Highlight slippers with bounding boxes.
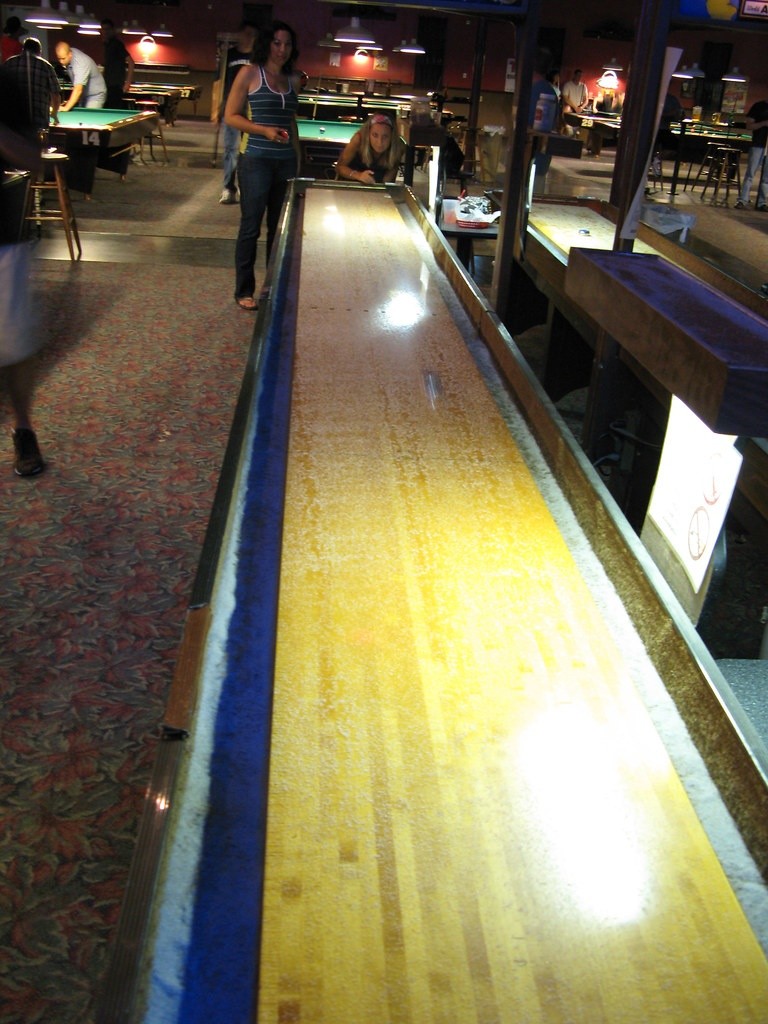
[235,293,257,310]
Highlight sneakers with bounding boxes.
[11,427,43,477]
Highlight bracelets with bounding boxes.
[350,171,356,180]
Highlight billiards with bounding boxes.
[691,129,694,132]
[320,127,325,133]
[704,131,707,134]
[617,116,620,119]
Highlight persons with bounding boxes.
[592,89,619,158]
[528,70,589,126]
[0,69,48,477]
[225,22,302,311]
[216,21,258,203]
[100,19,133,108]
[335,112,406,185]
[652,94,679,163]
[4,36,60,202]
[0,16,28,62]
[56,42,107,113]
[736,100,768,211]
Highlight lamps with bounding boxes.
[317,33,342,48]
[602,56,624,71]
[672,65,693,79]
[392,40,407,53]
[687,63,705,78]
[116,20,147,35]
[355,25,383,51]
[721,67,746,82]
[24,0,101,35]
[334,17,375,44]
[400,39,425,54]
[151,24,174,38]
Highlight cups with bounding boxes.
[342,84,349,93]
[336,83,342,92]
[712,113,722,123]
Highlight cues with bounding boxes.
[312,63,324,120]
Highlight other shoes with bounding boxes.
[218,188,235,205]
[651,153,662,178]
[733,199,751,209]
[754,205,768,212]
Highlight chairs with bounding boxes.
[433,137,481,196]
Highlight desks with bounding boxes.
[435,196,498,269]
[48,107,160,201]
[295,93,453,183]
[395,116,445,188]
[56,78,203,126]
[565,112,755,164]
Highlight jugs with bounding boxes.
[693,106,702,122]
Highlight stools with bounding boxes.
[121,98,170,166]
[25,153,82,264]
[683,142,741,206]
